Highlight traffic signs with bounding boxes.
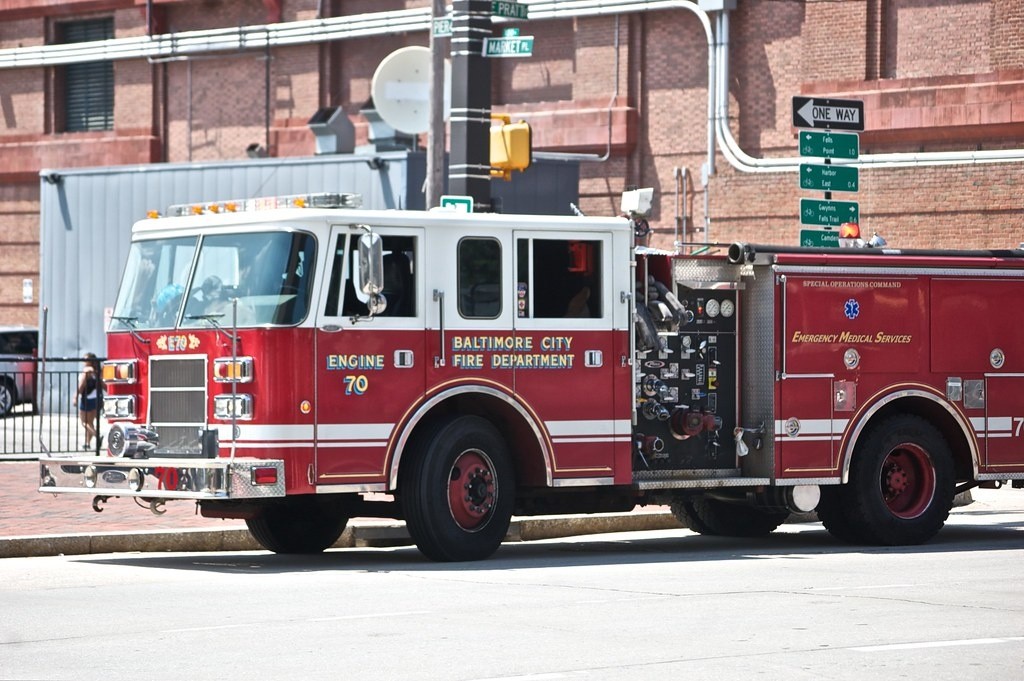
[793,97,865,248]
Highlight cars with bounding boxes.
[0,327,38,416]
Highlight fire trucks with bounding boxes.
[36,193,1024,566]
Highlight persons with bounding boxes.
[167,275,228,321]
[71,353,107,450]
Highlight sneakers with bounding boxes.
[99,435,104,447]
[83,444,90,451]
